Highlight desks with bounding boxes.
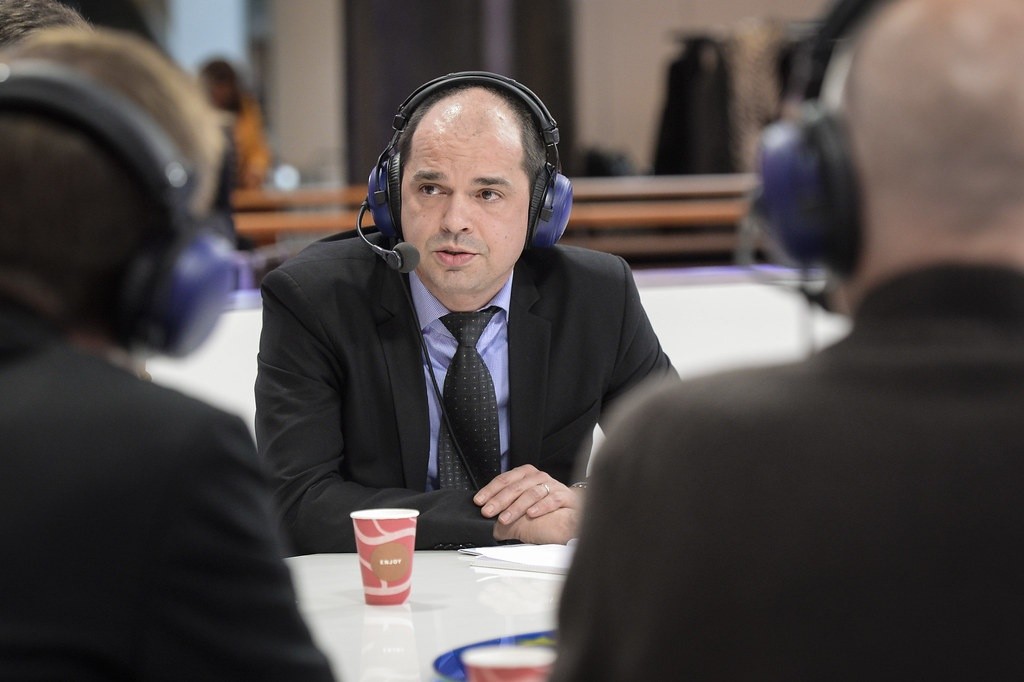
[284,551,566,682]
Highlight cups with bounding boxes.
[349,508,420,606]
[461,643,557,682]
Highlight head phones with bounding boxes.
[367,71,573,250]
[0,55,255,359]
[747,0,875,281]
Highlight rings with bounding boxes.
[537,483,549,493]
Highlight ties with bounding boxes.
[436,306,501,491]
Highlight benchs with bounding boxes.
[148,265,848,471]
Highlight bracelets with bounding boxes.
[579,485,587,488]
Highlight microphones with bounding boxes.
[737,226,829,313]
[357,196,420,273]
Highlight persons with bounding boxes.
[202,62,269,189]
[255,70,682,551]
[0,28,337,681]
[659,39,734,175]
[556,0,1024,682]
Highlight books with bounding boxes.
[469,538,578,576]
